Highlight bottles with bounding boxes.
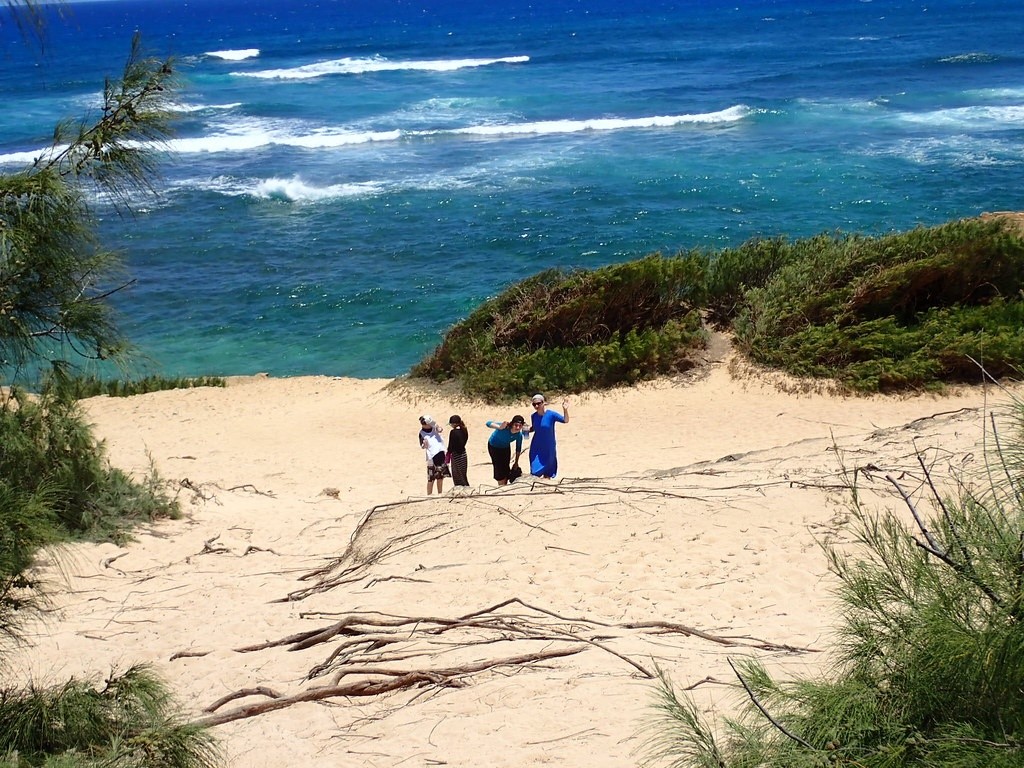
[445,454,450,463]
[523,428,529,439]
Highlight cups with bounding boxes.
[423,440,428,448]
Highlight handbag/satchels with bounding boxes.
[510,466,522,483]
[433,451,445,466]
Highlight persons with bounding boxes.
[525,394,570,478]
[418,415,452,495]
[444,414,469,486]
[485,415,525,486]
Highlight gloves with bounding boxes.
[446,452,451,463]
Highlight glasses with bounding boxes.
[532,401,542,406]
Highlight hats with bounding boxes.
[512,415,524,424]
[419,415,436,427]
